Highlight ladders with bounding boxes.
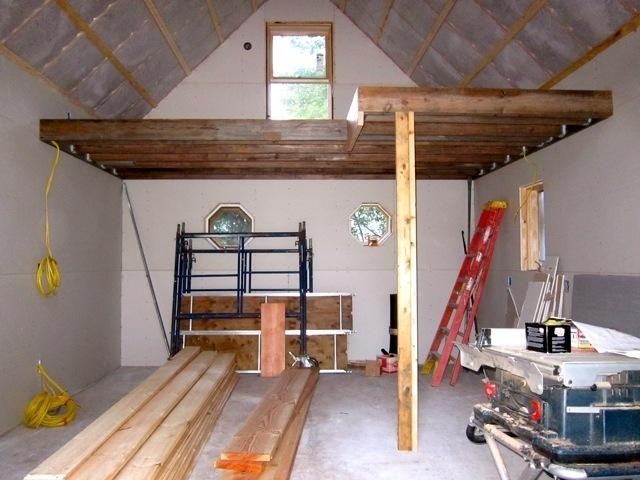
[419,201,508,388]
